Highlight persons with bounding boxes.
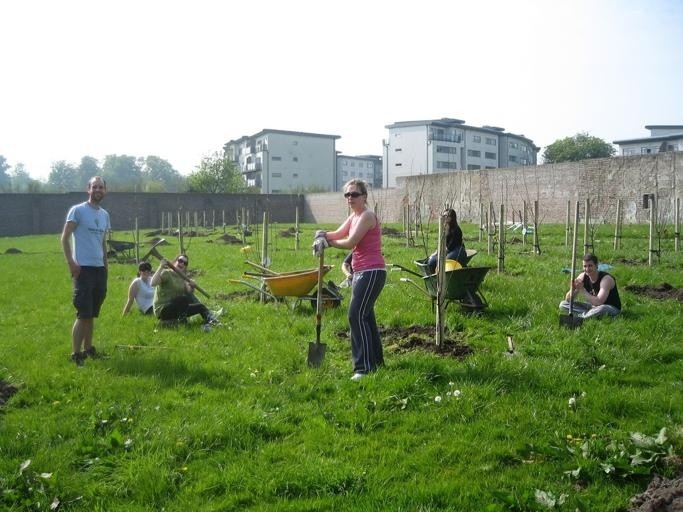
[61,176,111,364]
[340,251,354,288]
[149,255,223,325]
[122,261,156,316]
[431,208,467,268]
[312,178,386,380]
[559,252,621,320]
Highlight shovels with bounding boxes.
[307,233,327,369]
[558,200,584,330]
[501,333,520,361]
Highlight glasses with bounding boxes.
[178,258,188,266]
[345,192,363,198]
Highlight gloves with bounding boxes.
[313,231,329,257]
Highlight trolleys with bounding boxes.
[389,263,492,314]
[106,239,150,260]
[226,258,342,312]
